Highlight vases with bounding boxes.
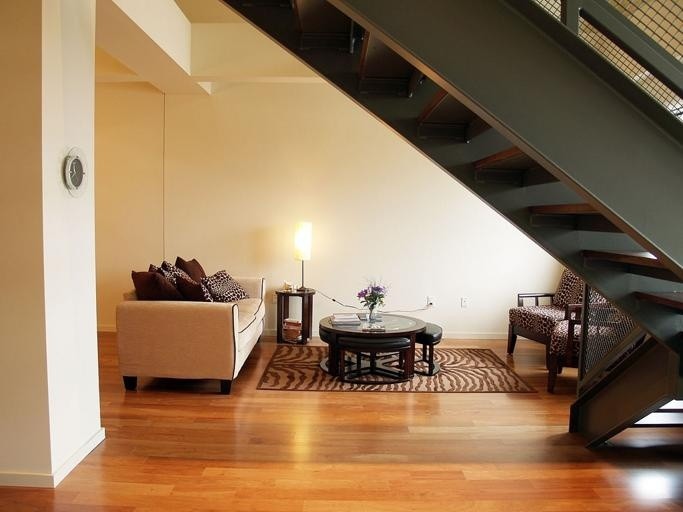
[366,309,376,323]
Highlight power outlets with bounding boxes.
[427,296,439,310]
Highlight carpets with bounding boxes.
[256,345,539,392]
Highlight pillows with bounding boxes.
[132,256,249,303]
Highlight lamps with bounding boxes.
[294,222,311,291]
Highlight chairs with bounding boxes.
[547,306,638,393]
[507,267,591,374]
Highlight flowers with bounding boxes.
[356,283,387,319]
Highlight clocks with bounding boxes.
[64,146,89,198]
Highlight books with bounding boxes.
[328,312,362,327]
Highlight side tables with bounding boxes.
[275,288,315,345]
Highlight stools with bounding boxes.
[339,336,411,384]
[396,323,442,376]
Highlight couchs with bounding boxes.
[116,278,265,395]
